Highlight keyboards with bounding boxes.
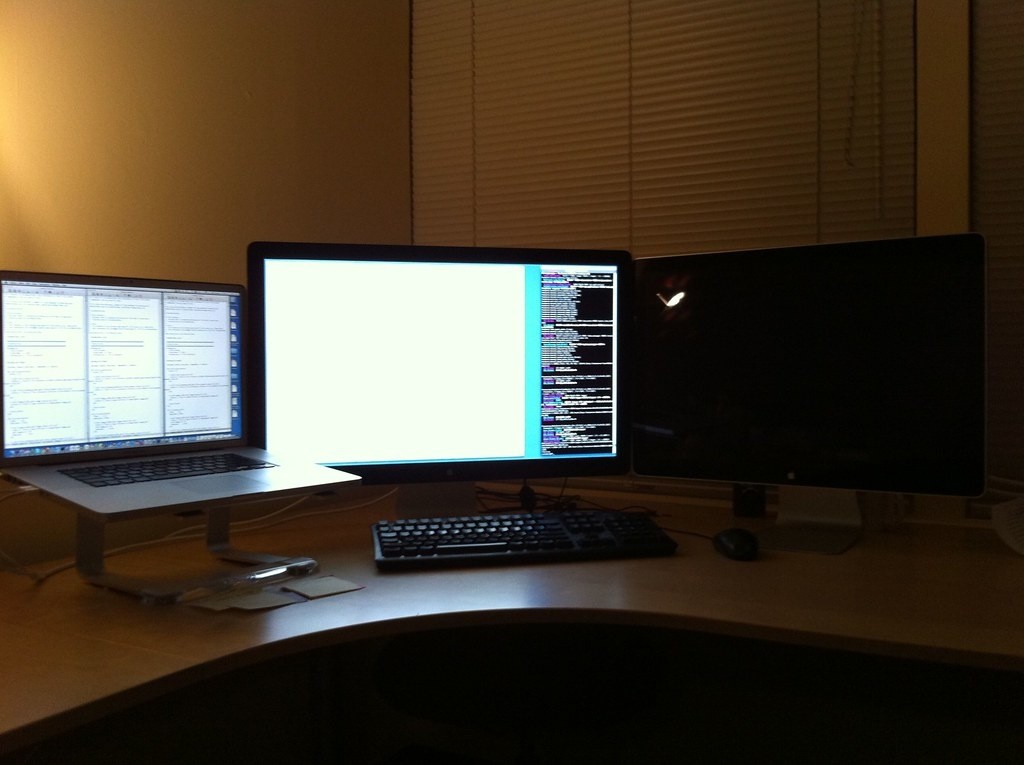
[371,513,675,568]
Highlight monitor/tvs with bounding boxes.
[245,240,634,520]
[627,233,988,557]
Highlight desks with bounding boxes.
[0,482,1023,754]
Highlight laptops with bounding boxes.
[0,270,360,524]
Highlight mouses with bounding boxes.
[713,528,760,560]
